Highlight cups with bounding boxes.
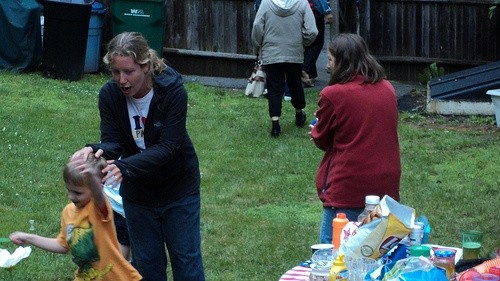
[311,249,376,281]
[462,232,483,260]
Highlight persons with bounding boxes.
[72,32,204,281]
[251,0,318,136]
[301,0,333,87]
[309,34,401,243]
[9,153,143,281]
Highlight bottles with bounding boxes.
[329,254,349,281]
[358,195,380,224]
[332,213,349,251]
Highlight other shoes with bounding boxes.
[271,119,280,137]
[300,71,316,88]
[295,109,307,127]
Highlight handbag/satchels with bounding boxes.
[245,67,265,99]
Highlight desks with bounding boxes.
[279,245,464,281]
